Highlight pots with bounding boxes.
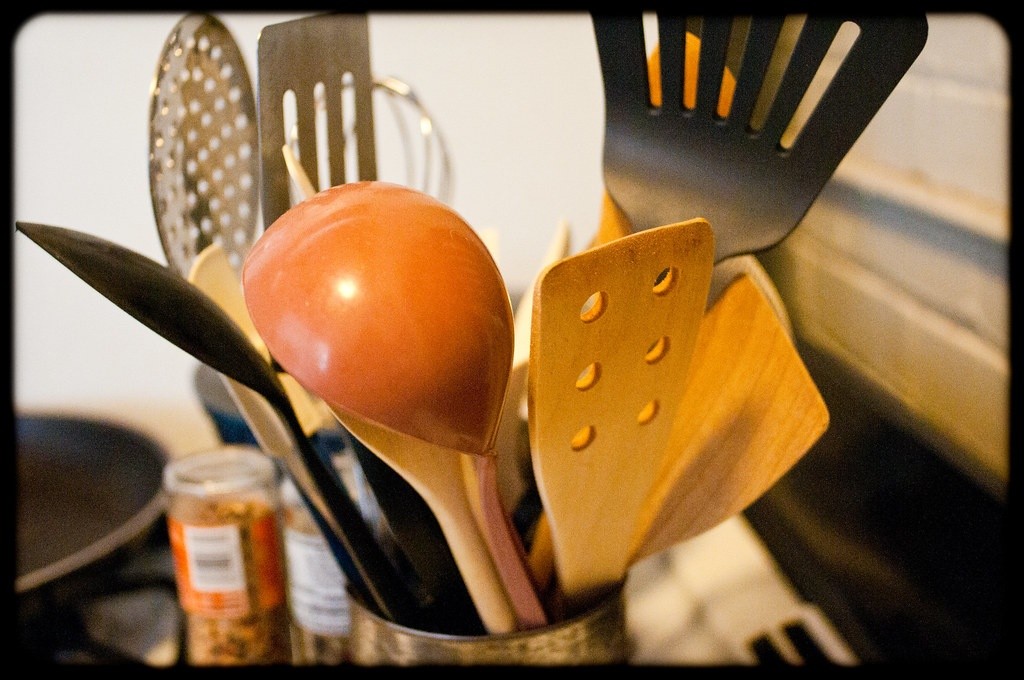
[17,415,169,666]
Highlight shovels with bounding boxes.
[12,9,930,637]
